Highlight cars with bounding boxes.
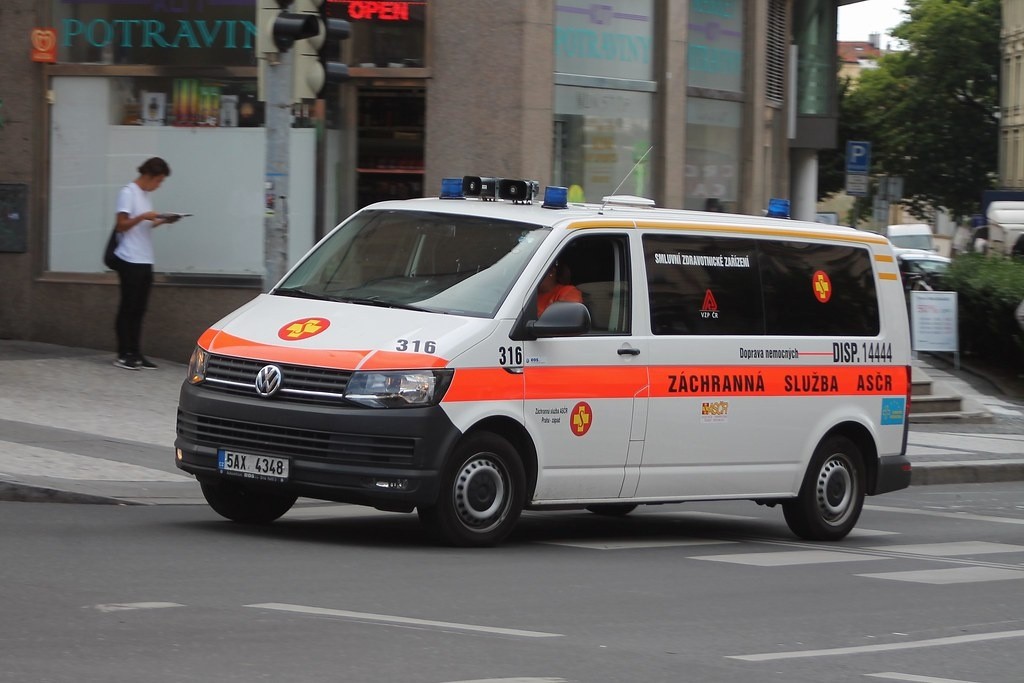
[896,253,951,301]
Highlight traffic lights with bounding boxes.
[297,14,351,131]
[255,0,319,63]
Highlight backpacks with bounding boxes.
[103,229,123,271]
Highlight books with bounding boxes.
[156,213,194,218]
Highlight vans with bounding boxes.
[174,177,913,540]
[887,224,938,254]
[986,201,1024,260]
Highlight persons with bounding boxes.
[114,157,181,370]
[537,260,583,317]
[702,197,722,213]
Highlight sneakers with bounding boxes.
[139,359,159,370]
[112,358,141,370]
